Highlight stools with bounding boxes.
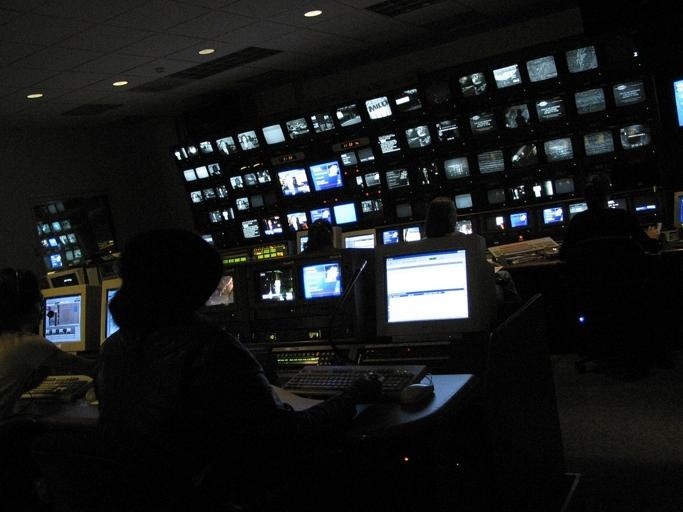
[1,412,50,512]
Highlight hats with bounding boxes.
[108,224,224,334]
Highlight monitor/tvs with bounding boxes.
[46,266,86,289]
[525,52,560,86]
[98,238,125,262]
[490,62,524,94]
[620,123,654,151]
[32,197,92,273]
[99,278,121,349]
[672,192,683,229]
[573,87,607,115]
[611,78,647,108]
[582,128,615,156]
[372,234,504,345]
[172,81,590,253]
[194,249,372,338]
[456,71,490,100]
[667,77,683,128]
[564,44,599,77]
[602,189,665,227]
[38,285,102,355]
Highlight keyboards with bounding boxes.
[20,374,94,402]
[486,237,561,260]
[282,364,428,402]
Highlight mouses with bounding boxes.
[400,383,434,409]
[85,387,96,401]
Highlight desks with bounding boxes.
[502,258,565,267]
[660,248,683,254]
[0,374,477,426]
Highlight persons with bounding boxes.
[0,267,98,512]
[559,175,660,261]
[298,219,349,258]
[417,196,513,306]
[95,225,381,512]
